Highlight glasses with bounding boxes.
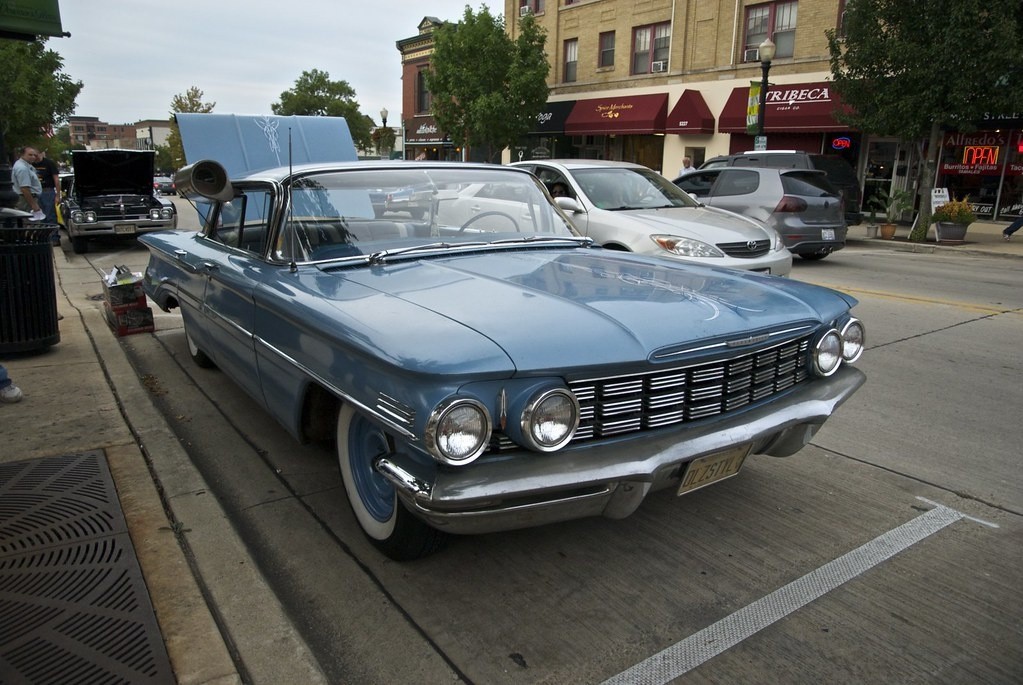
[551,190,566,196]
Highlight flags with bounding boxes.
[40,123,56,139]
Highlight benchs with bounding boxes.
[244,218,413,257]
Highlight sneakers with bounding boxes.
[0,384,24,404]
[1003,230,1010,241]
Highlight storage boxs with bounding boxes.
[102,301,154,337]
[102,272,146,310]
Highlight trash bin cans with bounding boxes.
[0,222,62,359]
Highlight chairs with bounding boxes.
[285,222,353,261]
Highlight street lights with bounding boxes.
[156,151,160,168]
[380,107,388,128]
[758,38,776,135]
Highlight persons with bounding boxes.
[11,146,42,223]
[32,146,61,247]
[0,364,22,403]
[552,181,569,199]
[596,177,628,208]
[1002,215,1023,241]
[678,157,696,178]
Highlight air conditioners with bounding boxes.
[744,48,761,63]
[651,60,668,72]
[520,6,531,16]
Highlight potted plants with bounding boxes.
[870,188,919,239]
[866,204,880,238]
[930,192,980,239]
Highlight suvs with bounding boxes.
[697,149,864,225]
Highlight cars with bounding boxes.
[135,111,867,562]
[645,167,847,261]
[154,176,177,196]
[436,158,792,278]
[55,173,74,228]
[61,149,178,255]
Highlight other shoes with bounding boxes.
[52,239,61,246]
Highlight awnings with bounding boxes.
[665,89,715,136]
[406,115,453,144]
[519,92,669,134]
[718,65,1023,137]
